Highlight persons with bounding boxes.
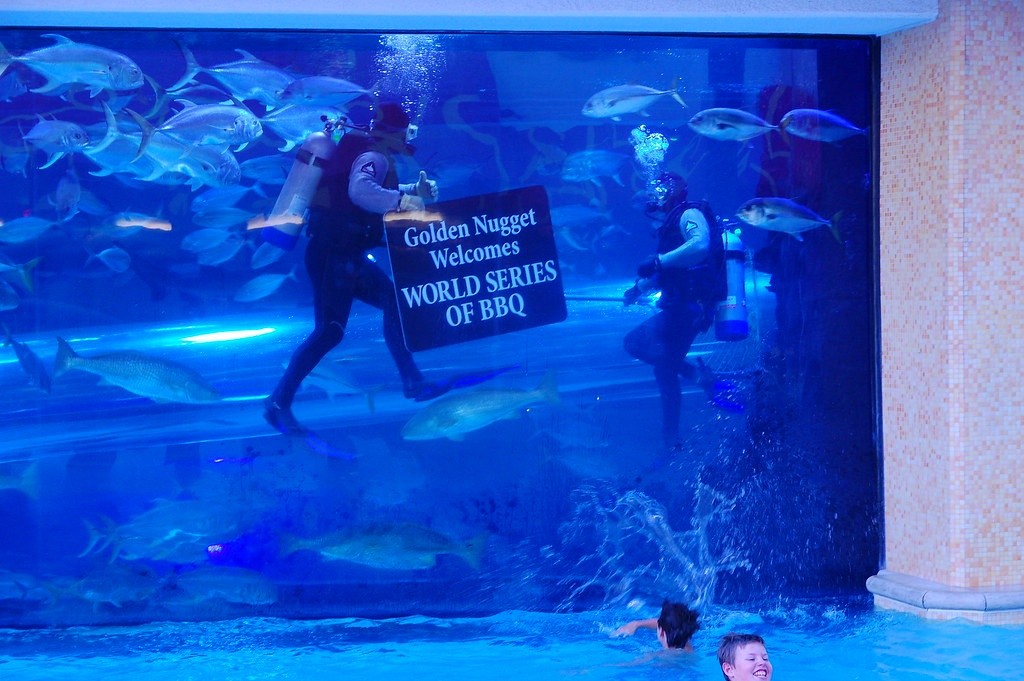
[623,172,729,453]
[263,103,447,433]
[717,633,772,681]
[615,601,700,652]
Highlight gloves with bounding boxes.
[624,285,642,307]
[417,171,439,203]
[399,193,425,216]
[637,255,662,281]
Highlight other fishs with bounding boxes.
[1,500,285,614]
[400,367,563,445]
[0,32,379,305]
[1,321,226,405]
[277,515,487,573]
[550,70,872,252]
